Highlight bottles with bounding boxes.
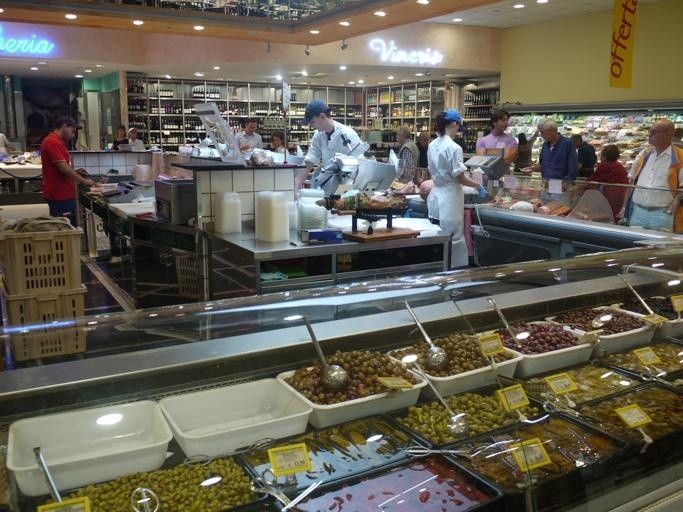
[128,100,147,111]
[149,133,196,143]
[134,80,144,93]
[151,102,195,114]
[157,86,173,98]
[219,104,279,115]
[230,120,242,130]
[464,93,490,105]
[129,118,204,131]
[464,107,492,118]
[228,88,238,99]
[193,88,220,99]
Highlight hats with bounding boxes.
[445,109,464,133]
[54,115,83,131]
[301,99,330,125]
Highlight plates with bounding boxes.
[41,454,268,512]
[600,336,683,380]
[672,335,681,343]
[236,413,432,494]
[559,379,683,458]
[657,372,682,392]
[516,359,653,413]
[440,412,631,508]
[236,495,287,511]
[278,448,503,511]
[384,383,553,450]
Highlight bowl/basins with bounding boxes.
[159,378,314,464]
[613,296,683,337]
[545,305,662,356]
[276,356,429,428]
[8,398,173,497]
[386,335,523,400]
[473,320,600,379]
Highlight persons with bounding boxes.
[536,119,577,180]
[517,126,538,167]
[235,117,262,151]
[393,127,420,183]
[294,99,360,199]
[577,144,628,219]
[416,131,430,169]
[272,132,290,155]
[570,134,597,177]
[128,128,145,151]
[40,116,95,228]
[476,110,518,166]
[616,119,683,231]
[113,125,128,150]
[427,109,480,270]
[0,132,9,154]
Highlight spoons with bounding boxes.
[592,311,613,329]
[303,315,347,392]
[414,362,470,437]
[404,300,448,371]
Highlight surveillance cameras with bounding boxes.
[341,43,348,50]
[304,49,311,56]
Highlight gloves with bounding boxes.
[478,185,488,199]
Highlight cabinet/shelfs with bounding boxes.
[462,83,499,120]
[460,121,491,152]
[399,172,683,262]
[498,102,683,178]
[2,242,683,511]
[366,82,444,157]
[127,78,363,152]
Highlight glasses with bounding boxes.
[647,129,657,136]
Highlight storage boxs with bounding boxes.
[153,180,194,224]
[0,284,87,362]
[171,247,212,300]
[1,219,83,293]
